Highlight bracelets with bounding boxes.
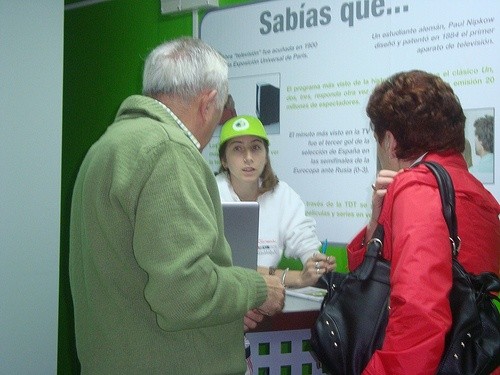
[361,238,366,247]
[282,268,289,284]
[269,266,275,275]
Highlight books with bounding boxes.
[286,285,327,302]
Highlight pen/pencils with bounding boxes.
[320,238,331,256]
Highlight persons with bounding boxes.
[467,115,494,184]
[346,69,500,375]
[214,115,337,289]
[69,36,287,375]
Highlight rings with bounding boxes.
[313,253,318,259]
[315,262,320,268]
[371,184,377,190]
[316,269,320,274]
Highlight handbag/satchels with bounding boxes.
[313,161,499,375]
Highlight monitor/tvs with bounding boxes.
[221,202,261,272]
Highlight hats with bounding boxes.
[217,115,269,151]
